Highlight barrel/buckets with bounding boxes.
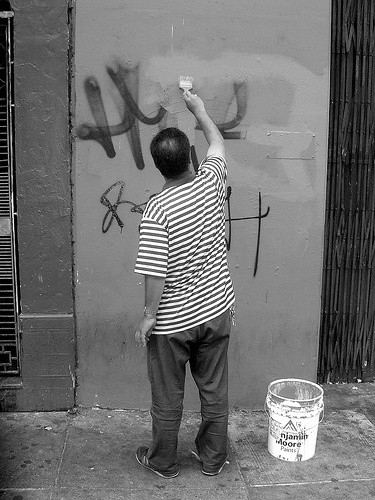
[264,378,325,463]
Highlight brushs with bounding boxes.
[178,75,195,96]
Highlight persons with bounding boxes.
[134,92,235,479]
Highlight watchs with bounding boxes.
[144,306,158,314]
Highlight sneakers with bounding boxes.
[202,457,229,475]
[135,445,179,478]
